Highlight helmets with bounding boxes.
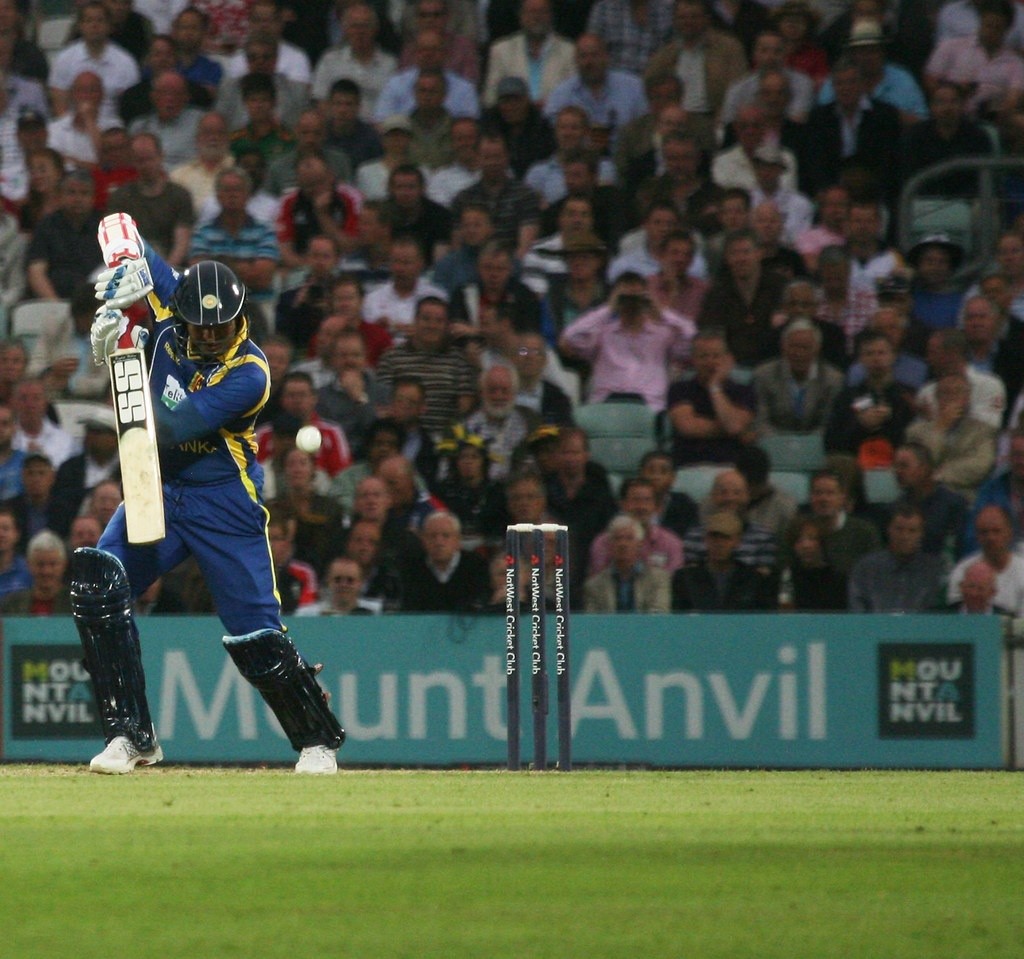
[172,261,246,327]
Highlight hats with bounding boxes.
[838,16,893,48]
[376,112,412,136]
[78,406,118,431]
[705,513,744,538]
[901,226,968,267]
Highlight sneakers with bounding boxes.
[293,743,340,776]
[88,723,165,773]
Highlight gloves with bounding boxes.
[89,301,151,370]
[96,257,154,310]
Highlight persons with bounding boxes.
[0,0,1024,618]
[68,213,346,775]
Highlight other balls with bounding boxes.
[296,426,322,453]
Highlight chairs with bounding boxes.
[571,403,829,516]
[909,196,976,262]
